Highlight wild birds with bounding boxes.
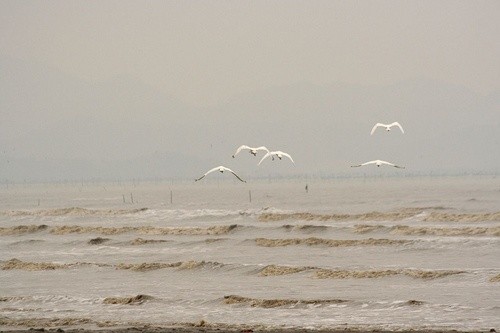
[370,121,405,134]
[194,166,247,184]
[232,144,296,167]
[350,159,406,170]
